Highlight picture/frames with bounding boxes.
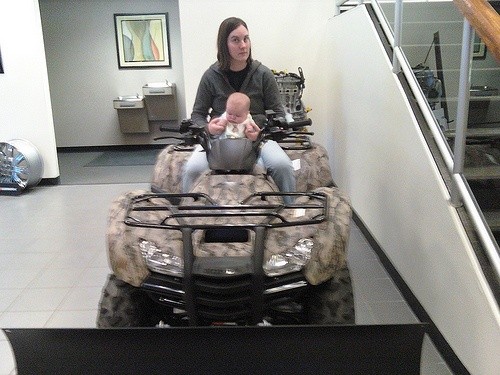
[114,12,172,69]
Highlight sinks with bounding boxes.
[142,83,175,95]
[113,96,144,109]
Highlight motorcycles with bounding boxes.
[95,68,357,328]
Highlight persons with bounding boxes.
[213,92,258,140]
[182,17,296,205]
